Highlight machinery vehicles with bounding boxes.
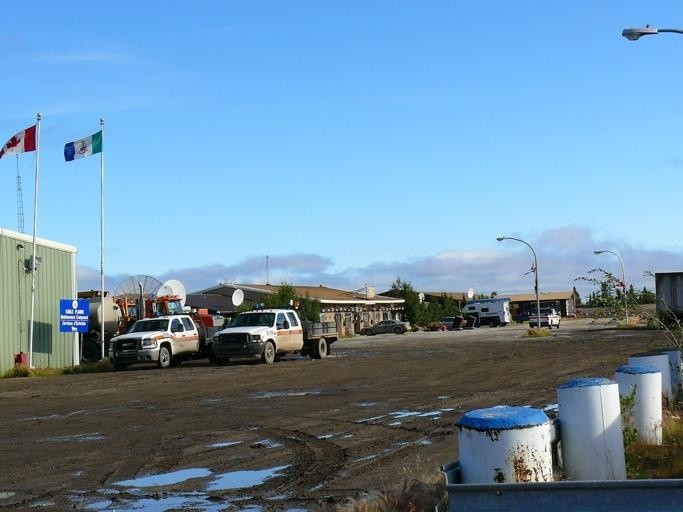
[77,291,225,368]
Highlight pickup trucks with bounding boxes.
[209,308,338,364]
[528,308,561,329]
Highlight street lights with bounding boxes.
[594,250,629,323]
[621,24,683,41]
[497,237,541,329]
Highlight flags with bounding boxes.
[0,125,37,158]
[64,130,102,161]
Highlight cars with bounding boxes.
[431,317,466,327]
[362,319,411,335]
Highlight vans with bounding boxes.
[461,298,511,328]
